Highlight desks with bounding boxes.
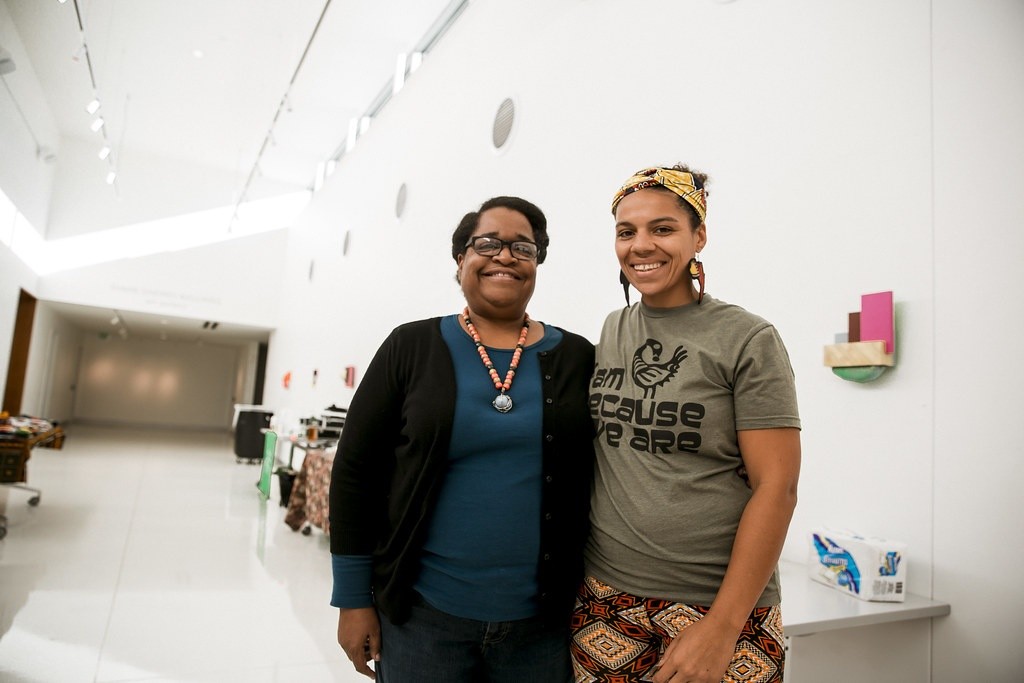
[779,560,951,683]
[0,412,67,538]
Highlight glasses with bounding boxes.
[465,235,541,261]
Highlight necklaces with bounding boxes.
[463,305,530,414]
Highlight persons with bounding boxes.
[568,165,802,682]
[331,196,594,683]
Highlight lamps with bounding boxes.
[90,117,104,132]
[72,44,88,61]
[106,172,116,184]
[85,99,101,115]
[99,145,111,160]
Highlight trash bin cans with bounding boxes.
[278,467,298,507]
